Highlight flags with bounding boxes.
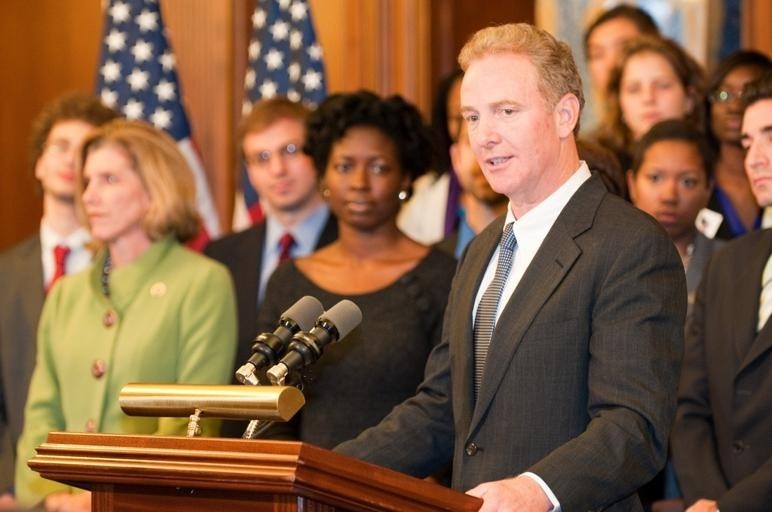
[93,0,220,254]
[232,1,328,239]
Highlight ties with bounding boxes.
[473,222,518,404]
[278,234,296,265]
[46,245,70,294]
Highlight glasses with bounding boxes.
[707,87,772,102]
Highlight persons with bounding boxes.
[330,22,688,512]
[699,51,768,239]
[14,118,238,512]
[600,37,707,148]
[264,91,458,489]
[394,65,464,222]
[0,95,126,512]
[673,77,766,512]
[584,5,663,101]
[400,109,511,257]
[201,98,338,438]
[623,119,727,312]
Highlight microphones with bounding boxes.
[266,300,362,384]
[235,296,325,383]
